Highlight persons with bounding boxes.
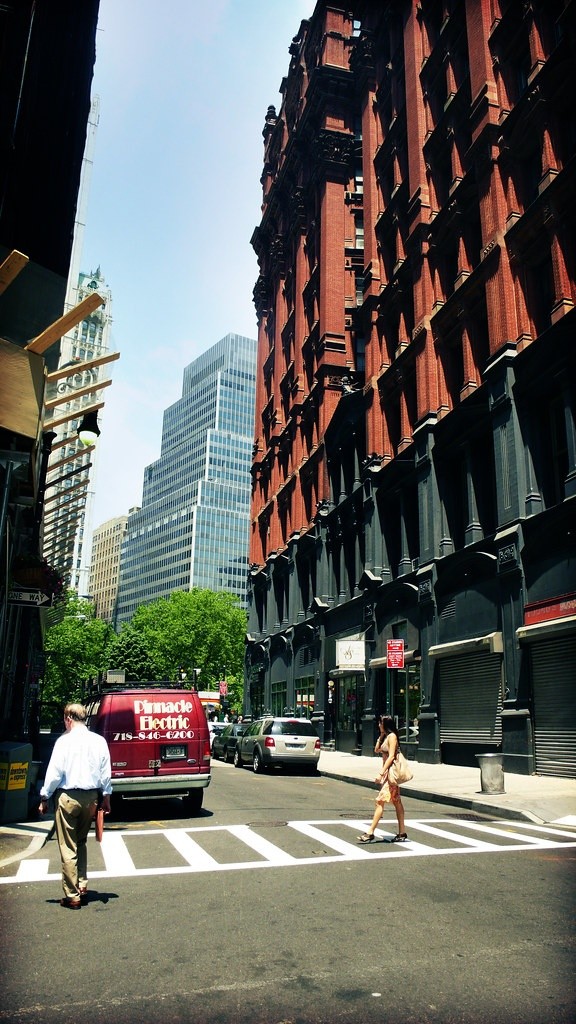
[237,716,243,724]
[38,702,113,909]
[223,714,229,723]
[356,715,408,842]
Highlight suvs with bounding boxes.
[81,669,211,819]
[234,718,321,774]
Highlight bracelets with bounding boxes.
[380,773,384,776]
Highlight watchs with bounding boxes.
[42,799,48,803]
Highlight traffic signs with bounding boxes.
[5,587,54,608]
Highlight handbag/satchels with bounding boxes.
[388,751,413,786]
[96,808,104,842]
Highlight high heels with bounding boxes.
[391,833,407,842]
[356,833,374,843]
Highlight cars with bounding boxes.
[208,722,251,763]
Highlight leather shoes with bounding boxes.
[79,887,87,895]
[60,898,81,909]
